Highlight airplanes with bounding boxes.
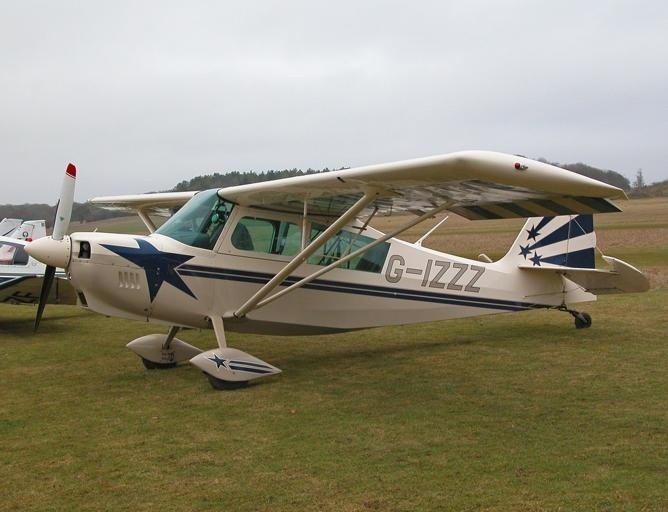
[1,216,76,307]
[1,148,654,390]
